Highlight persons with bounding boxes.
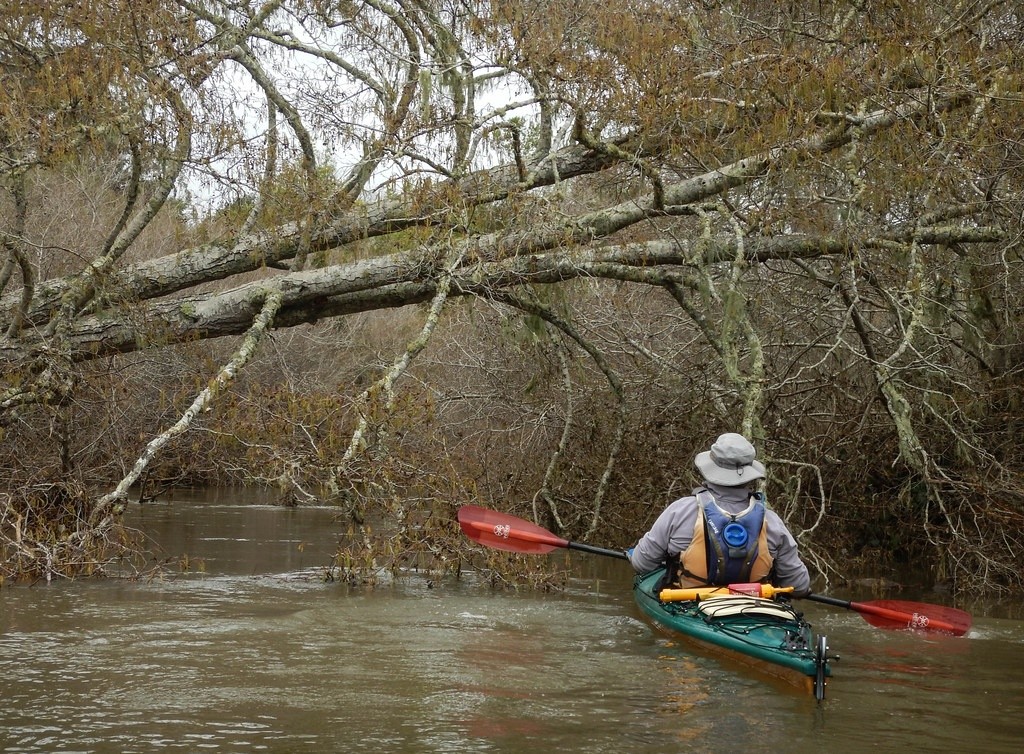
[626,431,811,607]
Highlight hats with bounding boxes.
[695,433,766,486]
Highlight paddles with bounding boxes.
[454,503,973,640]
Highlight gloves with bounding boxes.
[624,549,634,563]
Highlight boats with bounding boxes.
[632,566,844,702]
